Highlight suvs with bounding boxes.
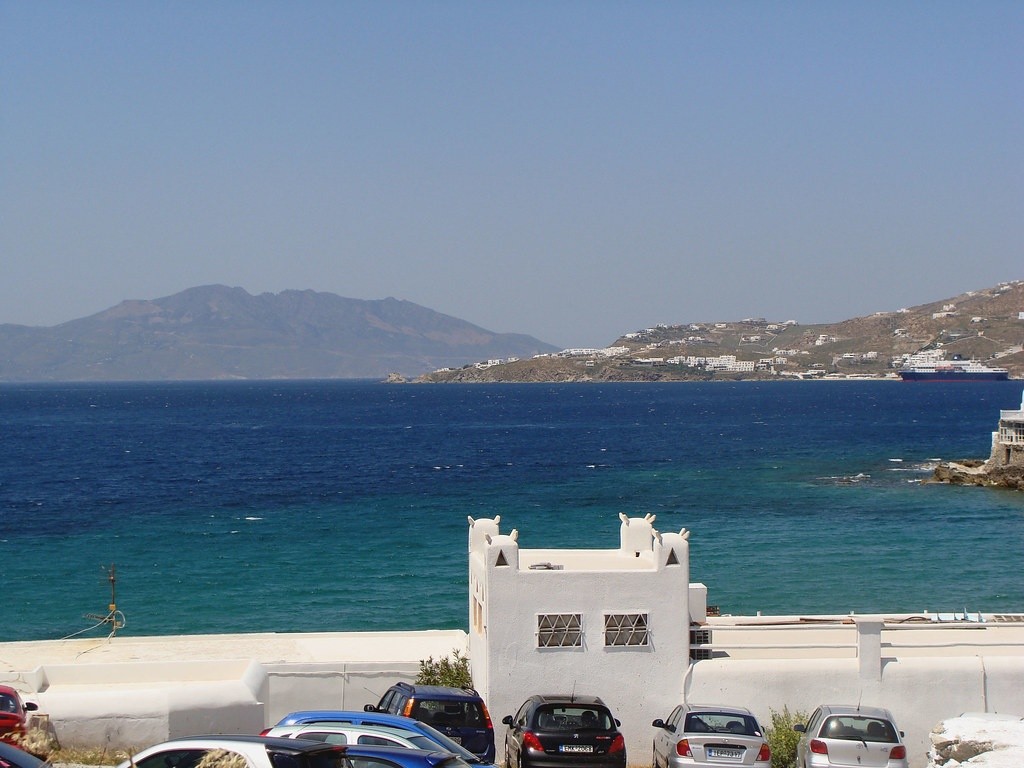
[363,681,496,764]
[502,693,627,768]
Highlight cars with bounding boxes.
[258,723,453,754]
[337,742,473,768]
[0,684,55,768]
[113,732,355,768]
[793,703,908,768]
[652,702,773,768]
[260,709,504,768]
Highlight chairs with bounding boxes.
[688,718,707,730]
[538,711,555,729]
[829,720,843,737]
[868,722,882,737]
[726,720,742,728]
[418,707,432,718]
[580,710,598,726]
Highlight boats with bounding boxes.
[897,354,1009,382]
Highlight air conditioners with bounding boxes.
[691,629,712,660]
